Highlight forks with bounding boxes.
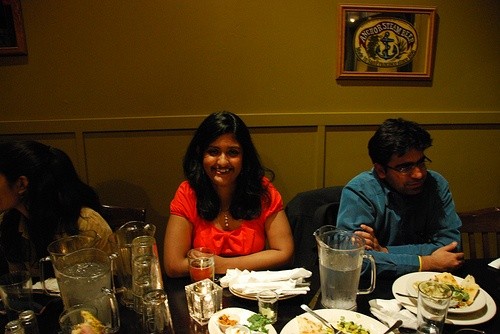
[300,304,347,334]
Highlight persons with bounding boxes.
[0,139,125,276]
[165,110,293,278]
[333,118,464,283]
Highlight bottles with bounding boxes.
[18,309,40,334]
[4,319,26,334]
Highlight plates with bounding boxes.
[422,288,486,314]
[208,307,277,334]
[228,281,307,300]
[391,272,497,325]
[279,308,395,334]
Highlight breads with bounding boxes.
[298,316,325,334]
[435,273,458,288]
[461,275,479,306]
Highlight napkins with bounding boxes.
[368,299,427,329]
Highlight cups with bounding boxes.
[58,304,98,334]
[416,280,454,334]
[0,270,33,314]
[140,289,175,334]
[187,247,216,284]
[131,235,164,315]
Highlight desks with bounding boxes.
[0,259,500,334]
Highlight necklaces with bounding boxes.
[220,210,230,227]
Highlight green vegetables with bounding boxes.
[243,314,271,334]
[337,321,371,334]
[439,284,469,300]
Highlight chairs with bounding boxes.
[286,186,343,268]
[101,205,146,233]
[457,208,500,258]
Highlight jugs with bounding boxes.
[313,225,377,311]
[38,229,102,297]
[108,221,157,309]
[52,248,121,334]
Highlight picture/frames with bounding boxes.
[335,3,436,81]
[0,0,28,56]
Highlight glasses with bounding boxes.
[386,155,433,174]
